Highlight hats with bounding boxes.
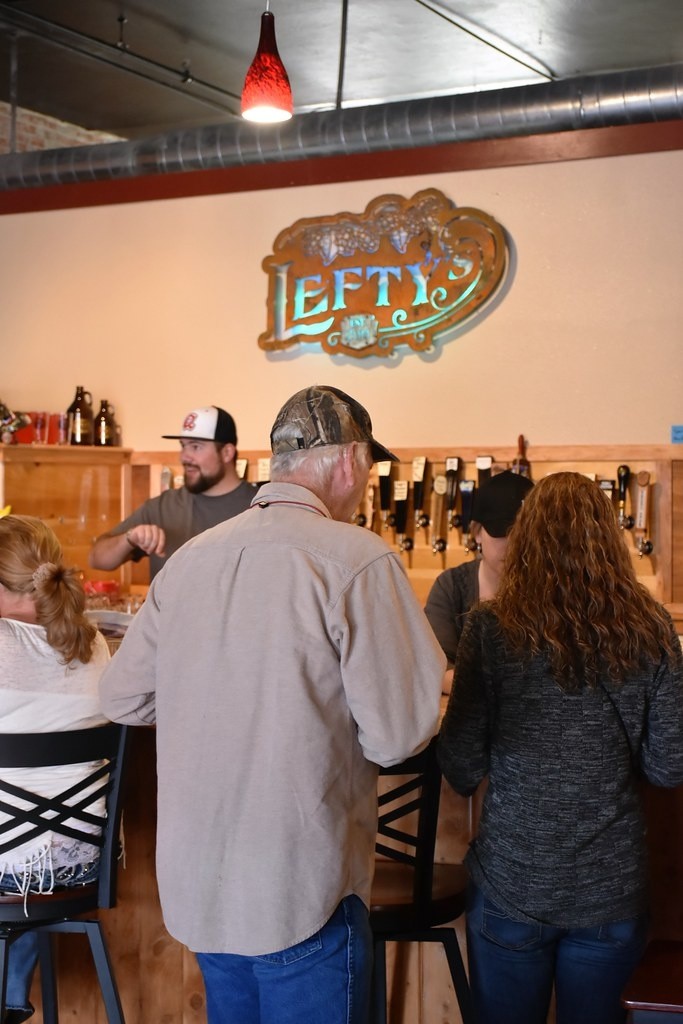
[160,405,238,447]
[269,385,401,465]
[470,470,537,538]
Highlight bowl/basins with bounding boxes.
[82,608,135,638]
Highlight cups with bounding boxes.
[14,409,61,445]
[81,589,144,616]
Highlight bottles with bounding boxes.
[65,385,94,446]
[94,398,115,448]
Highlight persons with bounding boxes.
[99,386,448,1024]
[0,514,125,1024]
[89,405,259,587]
[423,471,536,695]
[439,472,683,1024]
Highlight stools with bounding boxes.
[0,713,127,1024]
[354,729,499,1024]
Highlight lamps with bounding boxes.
[239,0,292,122]
